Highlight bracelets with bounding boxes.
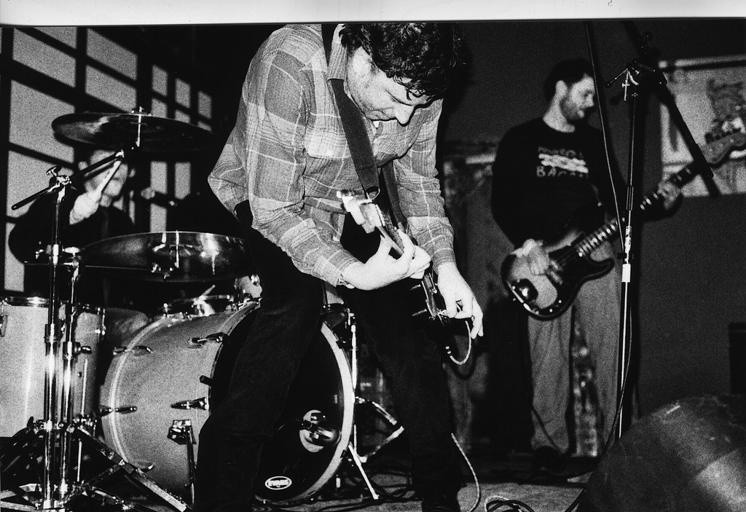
[522,241,538,257]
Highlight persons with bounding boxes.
[8,143,147,297]
[491,57,682,485]
[193,21,485,512]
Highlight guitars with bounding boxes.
[336,189,478,381]
[501,130,742,321]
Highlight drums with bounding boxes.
[97,304,356,511]
[0,289,105,437]
[578,394,742,512]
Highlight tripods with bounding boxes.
[0,144,192,512]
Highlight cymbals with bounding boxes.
[76,232,269,282]
[52,113,222,161]
